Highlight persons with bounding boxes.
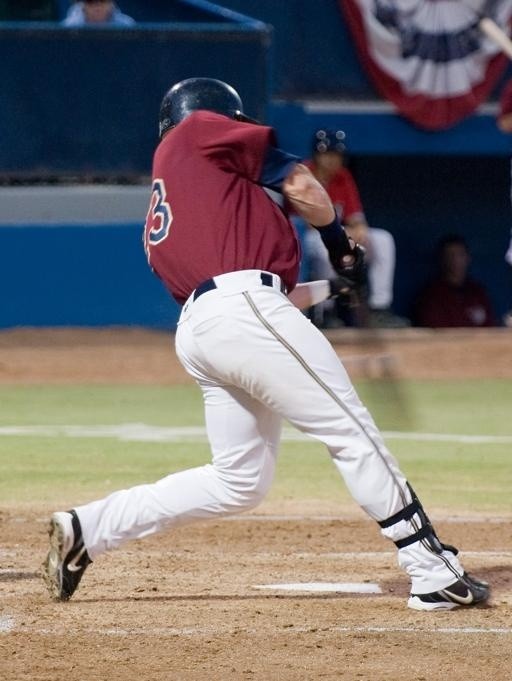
[282,128,411,329]
[413,233,493,327]
[59,2,156,184]
[496,75,512,133]
[46,76,494,611]
[500,242,512,327]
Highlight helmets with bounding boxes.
[157,76,261,141]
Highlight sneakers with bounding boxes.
[407,568,493,613]
[39,509,92,604]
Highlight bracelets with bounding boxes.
[311,207,341,241]
[309,279,331,305]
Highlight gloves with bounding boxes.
[327,276,372,306]
[324,226,366,276]
